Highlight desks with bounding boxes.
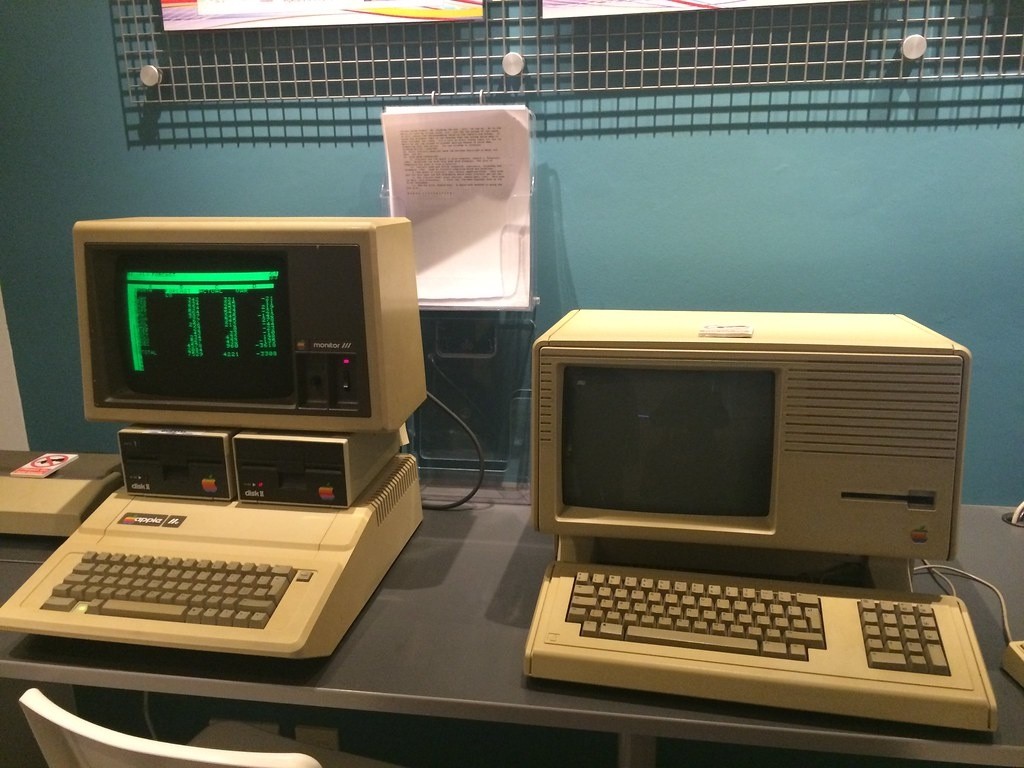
[0,485,1024,768]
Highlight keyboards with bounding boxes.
[37,549,314,631]
[566,573,951,677]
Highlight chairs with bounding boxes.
[18,687,323,768]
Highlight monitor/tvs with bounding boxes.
[558,365,776,518]
[108,249,292,403]
